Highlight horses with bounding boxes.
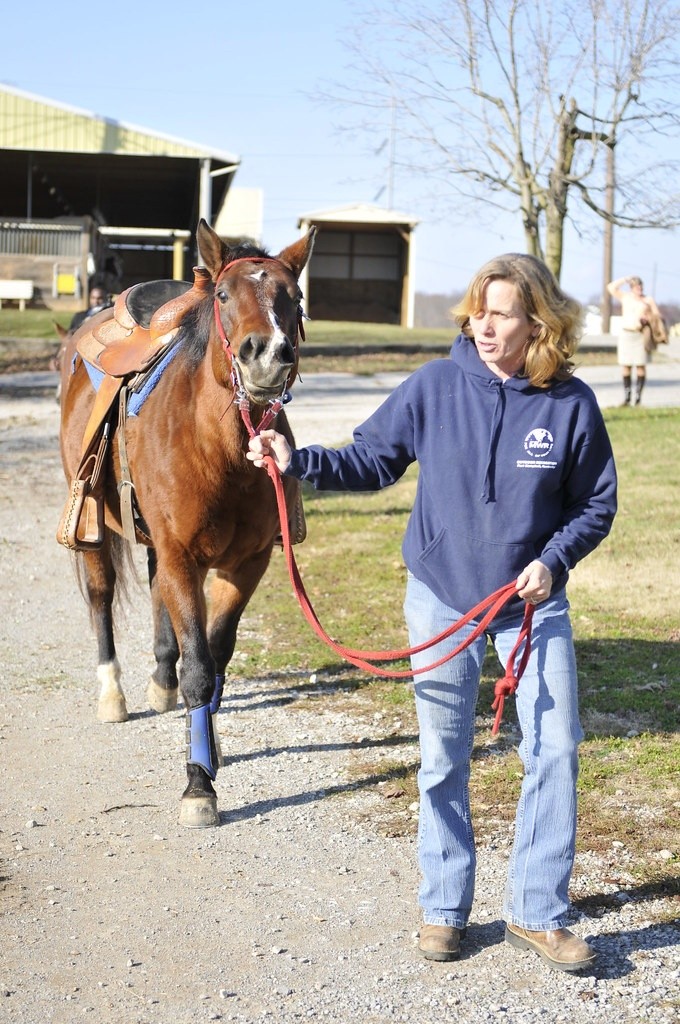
[58,222,322,826]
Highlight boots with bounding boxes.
[623,375,632,405]
[635,375,645,406]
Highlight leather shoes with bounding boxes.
[506,921,599,970]
[417,922,467,962]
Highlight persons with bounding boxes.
[244,252,618,975]
[70,271,120,334]
[606,274,668,405]
